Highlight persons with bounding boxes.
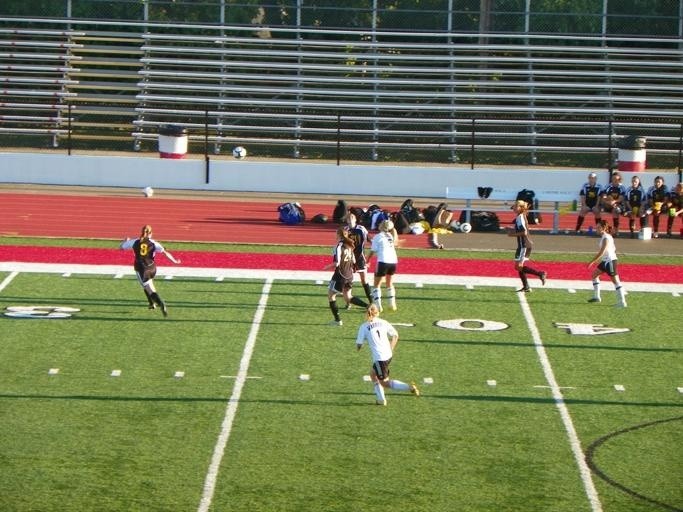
[575,173,683,238]
[506,200,545,293]
[355,303,420,407]
[586,220,627,309]
[120,225,180,317]
[326,213,398,326]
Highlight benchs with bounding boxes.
[445,186,611,234]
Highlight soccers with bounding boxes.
[450,220,460,231]
[233,146,247,160]
[460,222,472,233]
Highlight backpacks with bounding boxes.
[332,199,452,235]
[277,201,304,224]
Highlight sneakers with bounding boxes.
[538,270,546,285]
[408,381,419,396]
[613,302,626,307]
[375,400,386,405]
[665,234,670,238]
[587,298,601,303]
[652,231,658,238]
[615,233,624,237]
[328,319,342,326]
[517,287,532,293]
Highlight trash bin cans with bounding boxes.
[159,125,189,159]
[614,134,649,172]
